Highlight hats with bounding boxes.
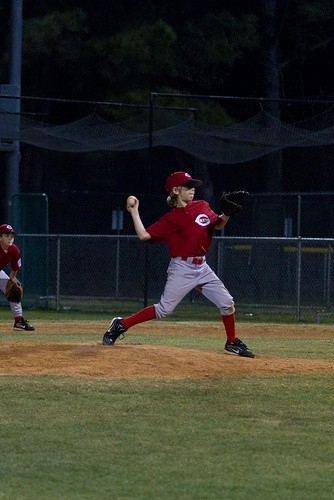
[0,224,16,235]
[165,171,203,192]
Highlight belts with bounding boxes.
[173,256,206,265]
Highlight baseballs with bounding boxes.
[128,196,137,206]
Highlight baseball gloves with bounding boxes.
[6,277,23,302]
[218,191,249,215]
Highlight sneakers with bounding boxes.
[103,317,127,345]
[13,317,35,332]
[224,337,255,358]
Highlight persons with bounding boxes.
[0,224,35,331]
[102,171,255,359]
